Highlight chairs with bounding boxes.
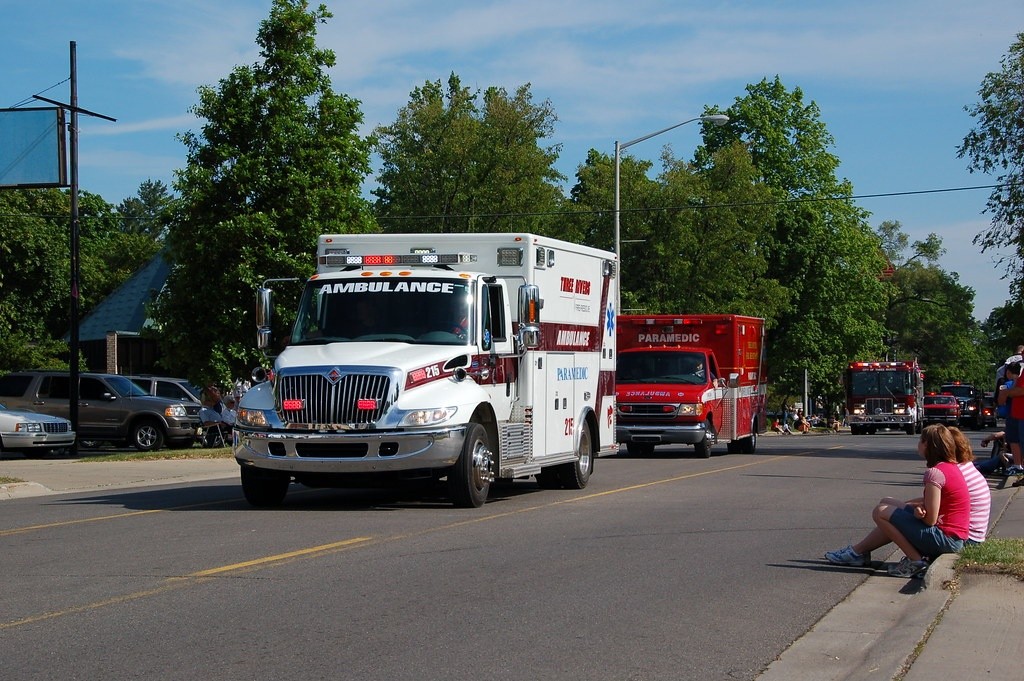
[201,422,227,448]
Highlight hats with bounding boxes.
[234,393,241,398]
[226,399,236,405]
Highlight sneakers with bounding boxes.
[824,544,871,566]
[888,557,930,578]
[1005,465,1024,476]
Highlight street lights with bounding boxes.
[615,112,729,313]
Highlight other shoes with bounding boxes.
[1012,478,1024,487]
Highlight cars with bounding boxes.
[982,394,999,427]
[0,403,77,460]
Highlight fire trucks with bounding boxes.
[939,381,984,430]
[843,356,925,435]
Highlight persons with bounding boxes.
[824,424,992,578]
[690,360,718,389]
[202,378,251,447]
[770,397,850,435]
[448,297,490,347]
[975,345,1024,487]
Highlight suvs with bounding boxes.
[922,394,964,430]
[1,371,204,452]
[115,374,206,408]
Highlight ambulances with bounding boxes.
[616,315,768,458]
[231,229,620,508]
